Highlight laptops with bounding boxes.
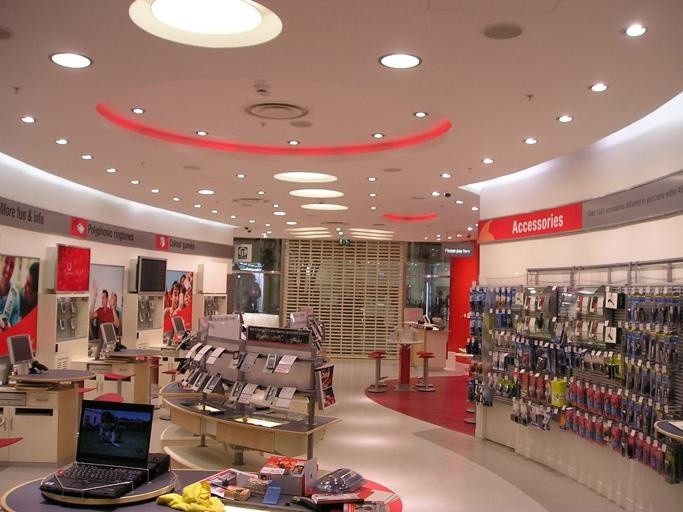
[41,400,154,498]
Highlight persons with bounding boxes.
[163,269,194,333]
[89,288,122,338]
[0,255,22,332]
[18,260,40,319]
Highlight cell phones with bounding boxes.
[292,465,304,474]
[262,487,280,505]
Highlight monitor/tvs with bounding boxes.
[7,334,34,364]
[171,316,186,333]
[100,322,118,344]
[135,256,167,295]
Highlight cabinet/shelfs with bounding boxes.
[158,334,343,466]
[463,278,682,485]
[0,344,196,467]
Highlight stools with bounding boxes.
[367,350,387,393]
[415,350,436,392]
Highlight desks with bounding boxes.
[0,467,258,512]
[386,339,423,392]
